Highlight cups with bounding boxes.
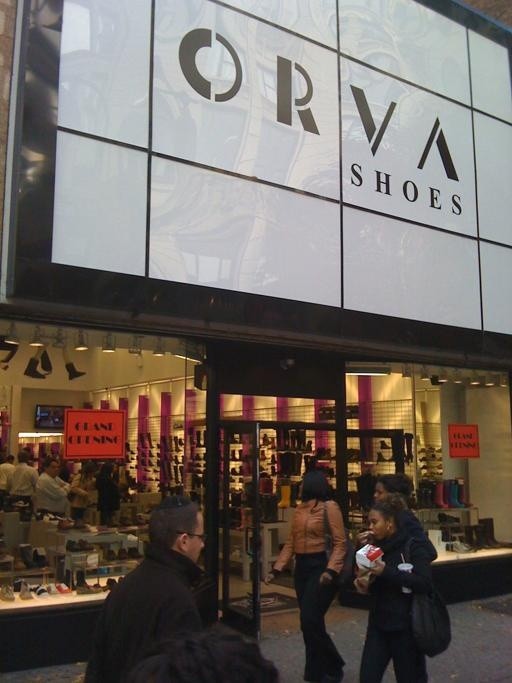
[397,562,416,595]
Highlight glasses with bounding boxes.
[175,531,207,541]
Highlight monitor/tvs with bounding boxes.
[35,404,73,429]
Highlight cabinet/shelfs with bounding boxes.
[0,574,132,673]
[338,507,512,610]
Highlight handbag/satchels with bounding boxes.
[322,507,354,584]
[410,587,452,661]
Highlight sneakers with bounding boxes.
[0,520,145,603]
[414,445,444,477]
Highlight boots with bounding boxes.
[24,351,52,380]
[124,427,414,509]
[66,362,86,381]
[436,513,504,552]
[414,478,471,510]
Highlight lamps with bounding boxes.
[402,366,507,387]
[5,321,200,363]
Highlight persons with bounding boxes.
[126,624,279,683]
[264,471,348,683]
[373,473,414,510]
[352,492,438,683]
[84,494,206,683]
[0,442,127,527]
